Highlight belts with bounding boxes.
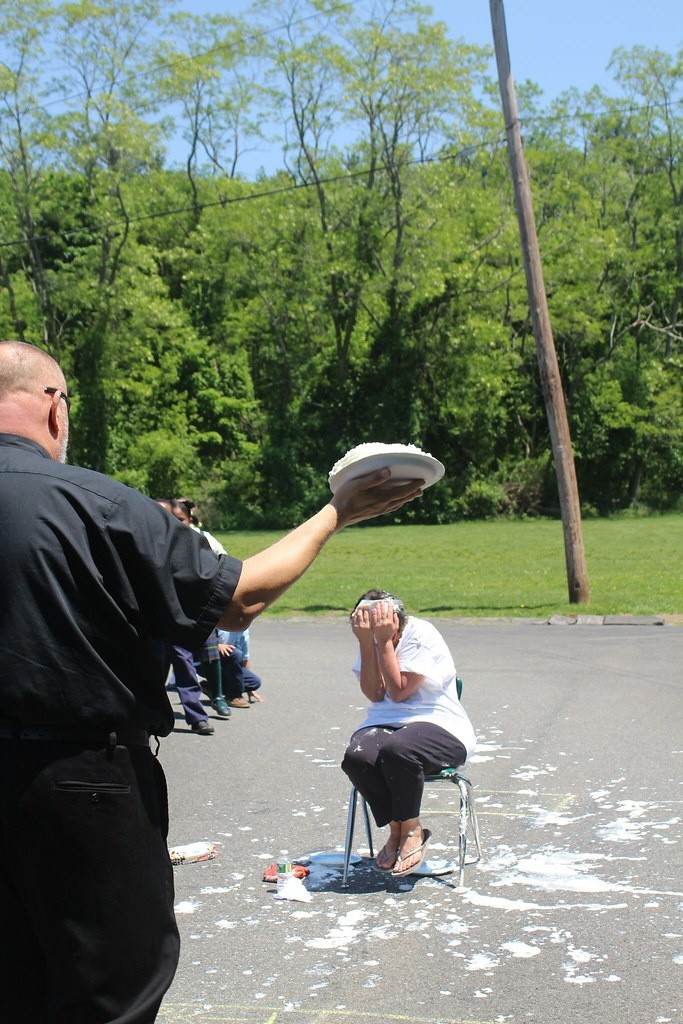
[0,720,149,746]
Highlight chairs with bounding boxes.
[340,677,481,889]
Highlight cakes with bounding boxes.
[328,442,435,483]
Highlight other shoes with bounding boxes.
[192,719,214,733]
[211,698,232,716]
[225,696,250,708]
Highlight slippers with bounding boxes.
[372,845,395,873]
[391,828,432,878]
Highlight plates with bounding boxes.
[411,860,455,875]
[309,853,362,865]
[330,452,445,495]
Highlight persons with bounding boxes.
[152,498,262,734]
[341,590,477,879]
[0,341,426,1024]
